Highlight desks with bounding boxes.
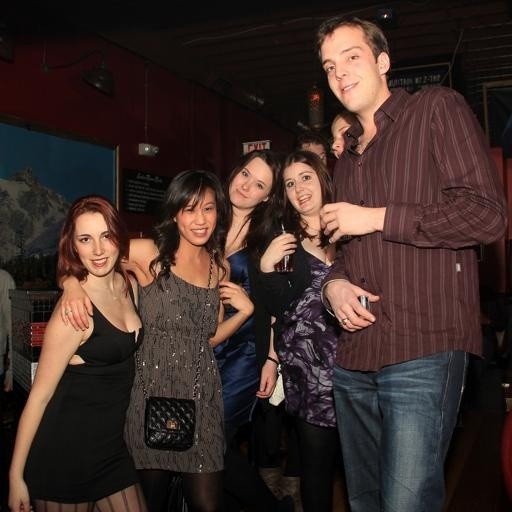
[9,288,62,393]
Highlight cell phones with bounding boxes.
[356,295,372,316]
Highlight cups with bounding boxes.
[273,230,296,273]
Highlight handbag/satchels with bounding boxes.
[142,394,197,453]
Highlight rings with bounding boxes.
[342,318,349,325]
[65,309,72,314]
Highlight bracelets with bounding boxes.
[267,356,278,364]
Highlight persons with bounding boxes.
[260,150,352,510]
[6,197,146,512]
[318,10,508,511]
[294,131,331,169]
[56,170,256,512]
[217,150,280,512]
[330,110,355,161]
